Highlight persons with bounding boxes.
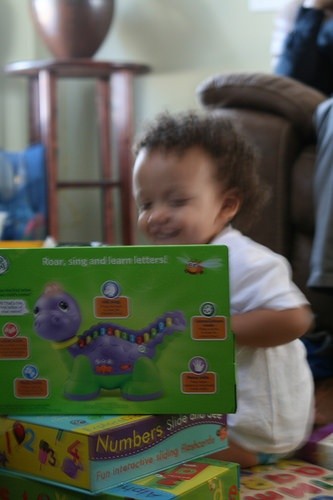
[129,107,318,467]
[278,1,333,290]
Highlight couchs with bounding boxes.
[201,72,333,329]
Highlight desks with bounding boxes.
[8,59,150,245]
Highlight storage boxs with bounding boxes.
[0,244,240,500]
